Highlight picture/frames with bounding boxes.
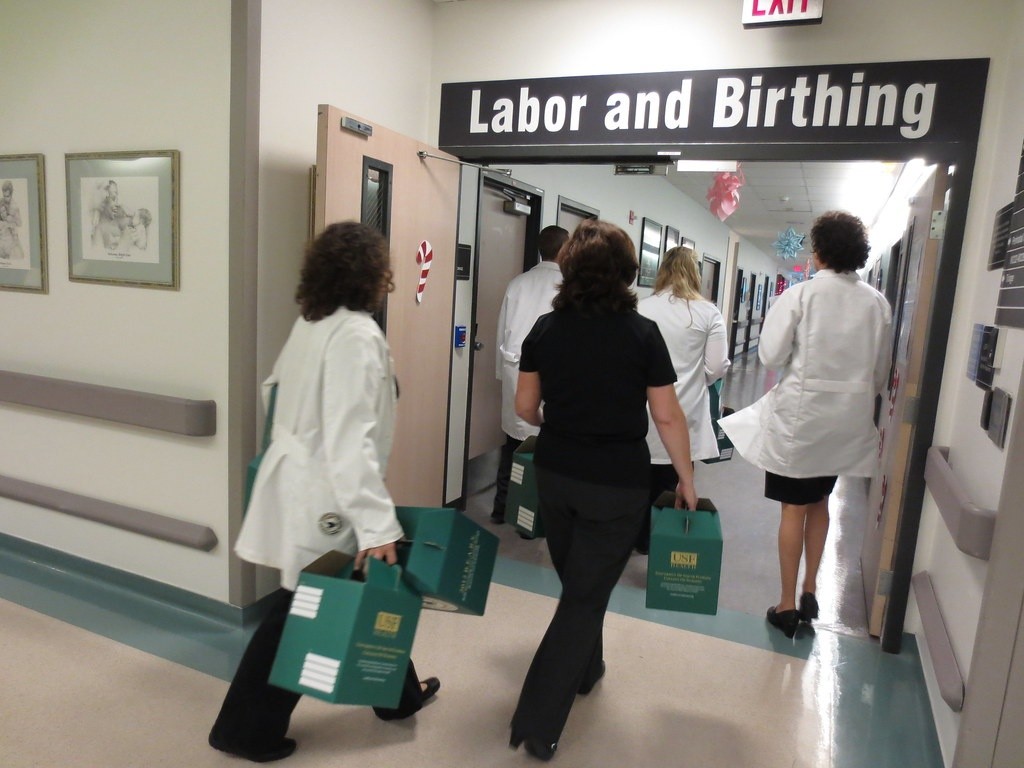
[637,216,696,288]
[64,148,182,293]
[0,152,50,296]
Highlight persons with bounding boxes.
[715,211,892,637]
[510,220,699,761]
[208,223,440,763]
[635,247,729,554]
[491,226,569,539]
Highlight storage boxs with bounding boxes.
[644,490,725,615]
[502,432,558,538]
[358,504,500,616]
[701,377,736,464]
[267,548,426,710]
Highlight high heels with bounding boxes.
[767,606,799,637]
[799,592,818,622]
[576,659,605,695]
[510,717,556,762]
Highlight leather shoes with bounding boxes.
[416,676,440,703]
[208,720,297,763]
